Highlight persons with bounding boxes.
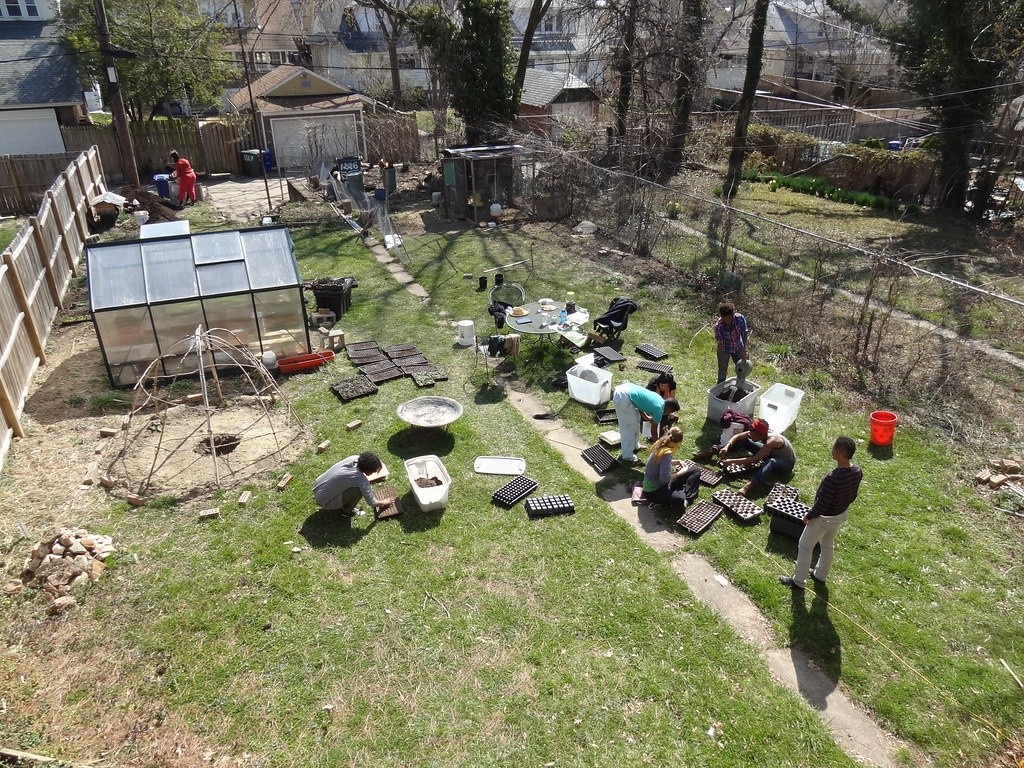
[613,373,680,466]
[778,436,864,589]
[716,418,797,495]
[313,452,395,517]
[641,426,701,508]
[714,303,751,384]
[170,149,198,207]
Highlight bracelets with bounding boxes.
[725,441,733,446]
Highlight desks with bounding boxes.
[506,302,588,354]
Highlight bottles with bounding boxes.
[561,309,567,323]
[566,292,576,313]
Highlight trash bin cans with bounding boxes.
[152,174,170,198]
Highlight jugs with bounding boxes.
[734,358,753,390]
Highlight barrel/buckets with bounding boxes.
[490,203,501,216]
[456,319,475,345]
[720,422,744,446]
[375,189,385,201]
[869,411,897,447]
[479,277,487,289]
[348,171,365,200]
[495,274,504,285]
[432,192,441,207]
[133,211,149,227]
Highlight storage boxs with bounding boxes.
[405,455,451,512]
[566,364,614,406]
[706,377,761,425]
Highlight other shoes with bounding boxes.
[640,445,647,450]
[778,574,804,592]
[622,455,638,462]
[340,507,365,518]
[809,568,825,585]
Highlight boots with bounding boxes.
[693,445,720,461]
[738,478,759,497]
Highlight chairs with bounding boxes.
[475,333,522,381]
[591,296,638,345]
[487,284,527,336]
